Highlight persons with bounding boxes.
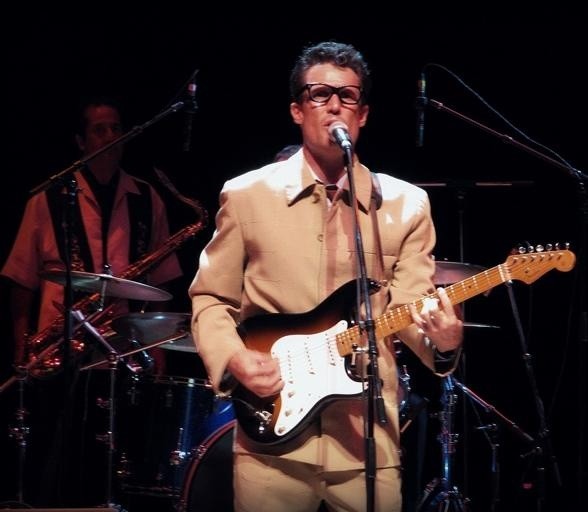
[187,40,464,512]
[0,95,184,503]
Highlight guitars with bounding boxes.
[232,242,577,444]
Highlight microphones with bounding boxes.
[327,121,352,150]
[417,71,426,148]
[183,79,196,151]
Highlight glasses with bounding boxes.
[294,83,363,105]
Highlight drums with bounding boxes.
[396,365,410,415]
[164,402,238,511]
[124,341,211,388]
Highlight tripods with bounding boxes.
[415,376,467,512]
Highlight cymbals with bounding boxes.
[112,310,198,353]
[430,261,488,286]
[36,269,173,302]
